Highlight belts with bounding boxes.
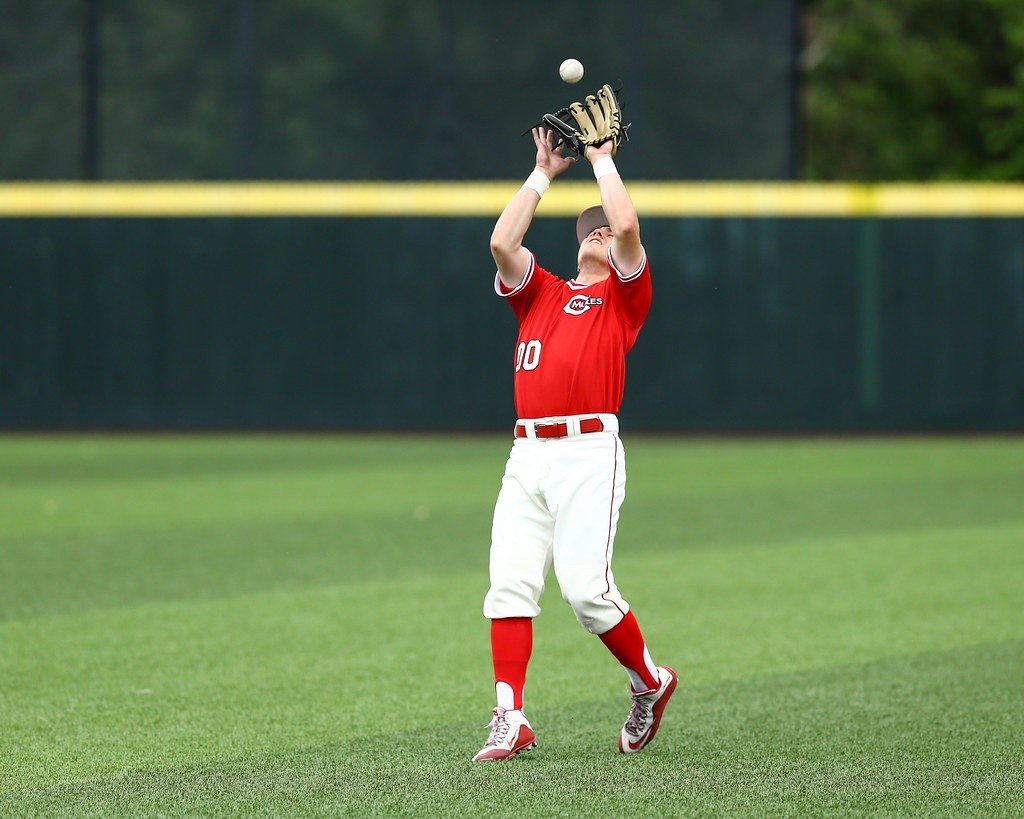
[515,418,603,438]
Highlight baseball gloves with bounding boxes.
[520,77,632,163]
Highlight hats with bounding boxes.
[576,205,609,245]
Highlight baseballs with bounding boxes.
[558,58,584,84]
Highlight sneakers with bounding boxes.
[472,708,536,761]
[618,665,679,754]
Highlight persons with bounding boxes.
[467,84,677,763]
